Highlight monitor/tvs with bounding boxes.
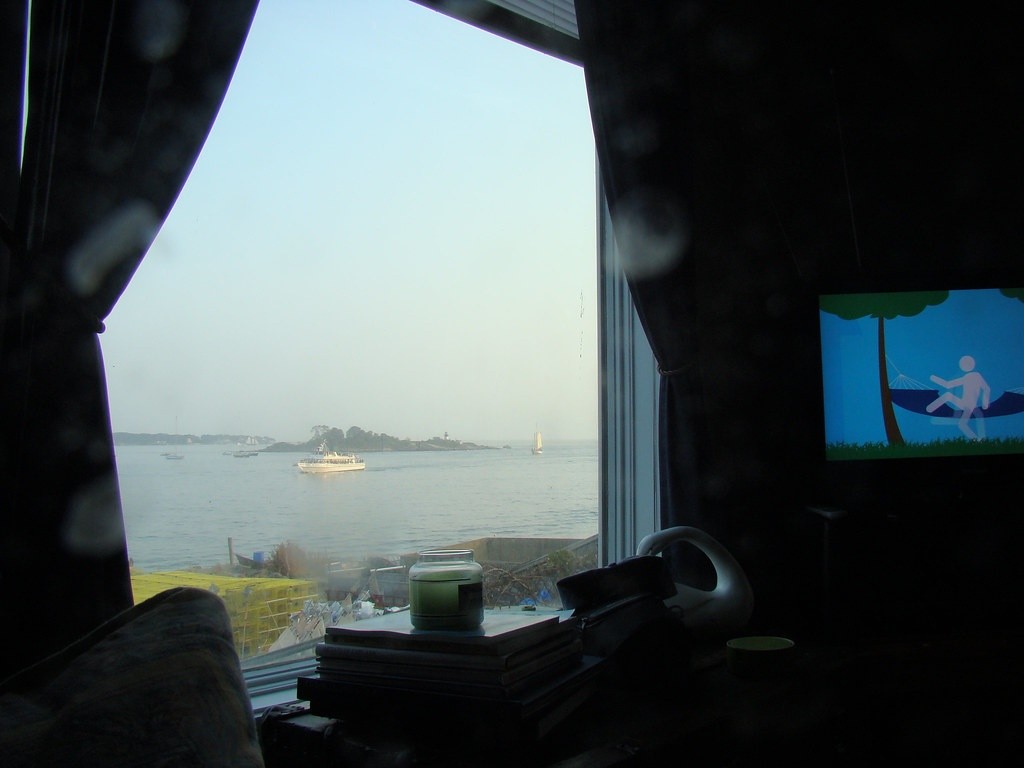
[810,279,1024,470]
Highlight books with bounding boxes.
[288,607,615,762]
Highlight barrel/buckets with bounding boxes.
[253,551,264,563]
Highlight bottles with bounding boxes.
[408,549,484,632]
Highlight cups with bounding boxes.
[727,637,798,686]
